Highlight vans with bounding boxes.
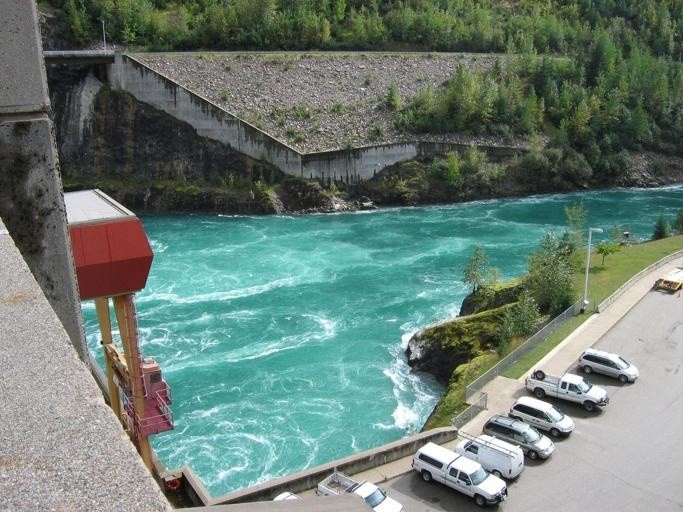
[507,395,576,438]
[578,350,639,383]
[480,411,555,461]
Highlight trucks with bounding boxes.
[454,435,526,481]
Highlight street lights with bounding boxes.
[580,224,602,314]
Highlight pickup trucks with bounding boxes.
[316,472,406,511]
[523,368,609,412]
[410,442,509,507]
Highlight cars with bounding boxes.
[271,492,302,500]
